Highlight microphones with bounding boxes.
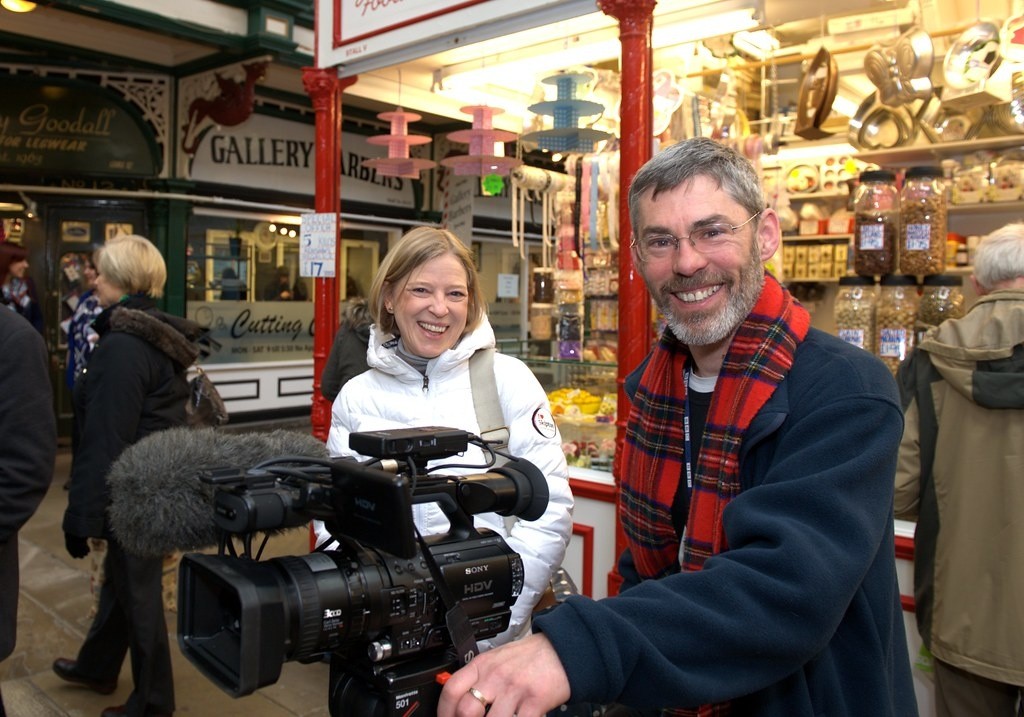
[103,427,405,558]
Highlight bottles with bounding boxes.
[872,276,920,377]
[896,165,946,274]
[854,168,901,274]
[834,275,875,356]
[528,267,553,383]
[917,275,966,326]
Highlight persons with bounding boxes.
[314,228,574,651]
[0,239,50,717]
[219,267,247,300]
[51,235,212,717]
[321,296,377,402]
[265,266,294,301]
[894,224,1024,717]
[62,243,105,490]
[437,135,921,717]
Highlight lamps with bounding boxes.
[431,0,762,95]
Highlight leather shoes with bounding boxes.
[53,658,116,694]
[100,705,129,717]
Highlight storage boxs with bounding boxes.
[784,244,851,280]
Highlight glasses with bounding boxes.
[629,209,763,263]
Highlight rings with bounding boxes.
[469,688,487,706]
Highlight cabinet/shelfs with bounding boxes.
[779,133,1024,284]
[584,295,619,334]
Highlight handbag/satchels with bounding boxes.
[530,568,580,616]
[184,371,229,431]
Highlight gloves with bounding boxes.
[64,533,90,560]
